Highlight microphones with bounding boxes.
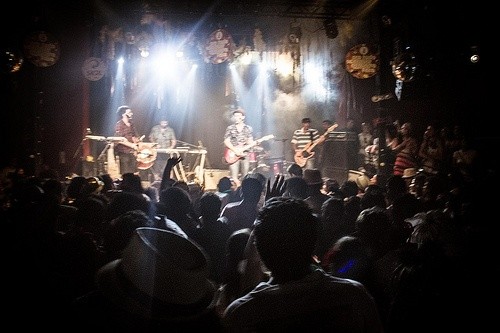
[87,128,92,134]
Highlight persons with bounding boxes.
[292,118,325,169]
[113,106,139,174]
[223,109,262,179]
[149,118,176,178]
[0,155,500,333]
[317,112,500,177]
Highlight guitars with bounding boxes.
[294,123,340,168]
[224,133,275,163]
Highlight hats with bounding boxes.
[304,168,323,185]
[301,118,311,123]
[355,176,370,190]
[402,168,420,178]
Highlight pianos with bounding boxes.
[157,146,208,156]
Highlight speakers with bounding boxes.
[202,169,231,191]
[323,140,359,183]
